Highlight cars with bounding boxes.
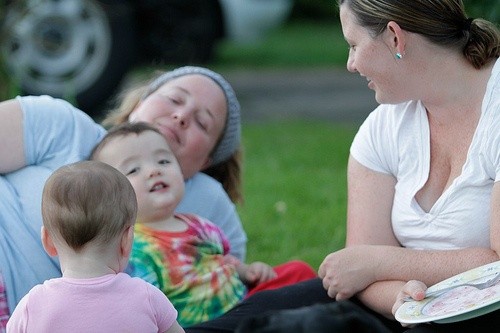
[0,0,231,118]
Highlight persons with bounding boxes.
[182,0,499,333]
[0,66,241,333]
[5,160,186,333]
[88,121,317,330]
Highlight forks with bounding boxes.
[403,274,500,301]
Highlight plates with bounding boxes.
[394,261,500,324]
